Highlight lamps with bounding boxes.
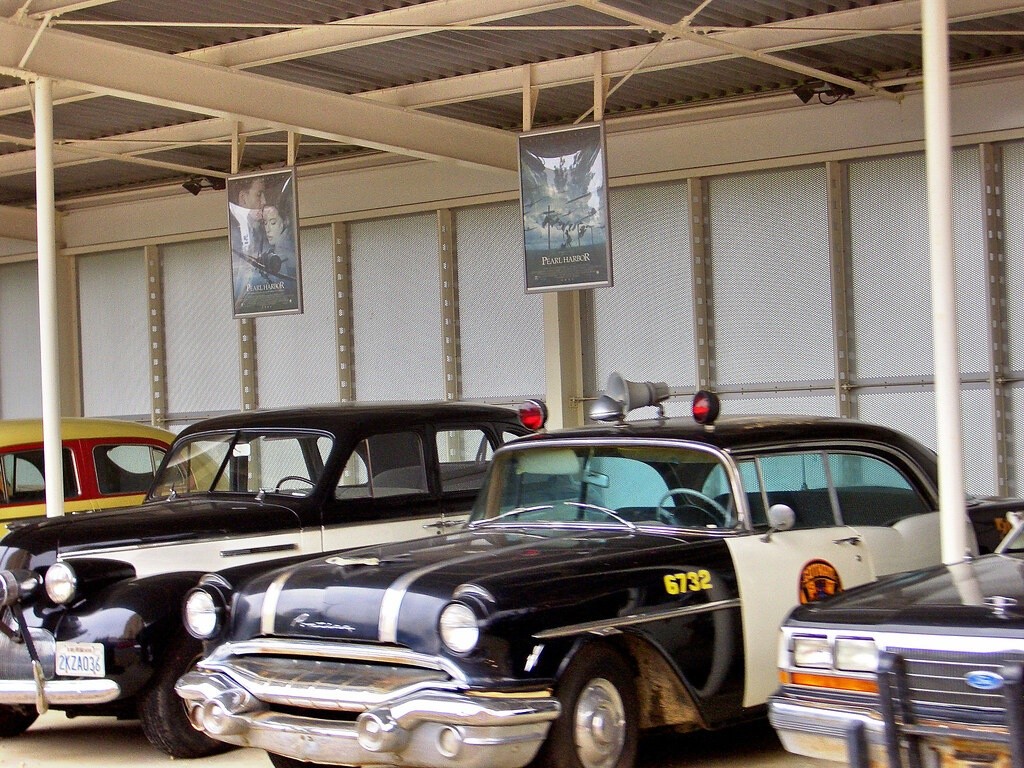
[793,77,856,105]
[182,165,226,196]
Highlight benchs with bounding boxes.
[366,460,486,492]
[705,491,928,526]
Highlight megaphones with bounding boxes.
[607,372,670,416]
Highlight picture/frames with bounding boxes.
[516,120,616,294]
[226,164,305,319]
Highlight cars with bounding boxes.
[176,388,1024,768]
[0,399,603,758]
[0,418,230,544]
[767,508,1024,768]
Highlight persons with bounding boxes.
[228,176,296,284]
